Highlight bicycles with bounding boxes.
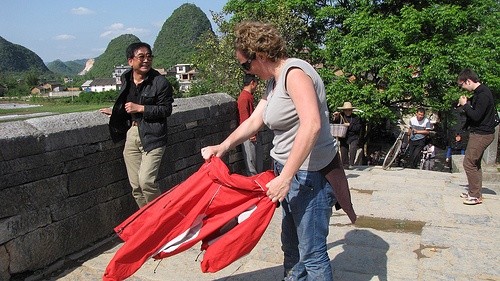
[383,120,432,170]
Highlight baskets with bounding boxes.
[330,113,348,137]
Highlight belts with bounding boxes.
[131,122,138,126]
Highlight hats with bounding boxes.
[337,102,356,109]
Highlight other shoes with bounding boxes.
[406,163,416,169]
[448,170,452,173]
[343,164,354,170]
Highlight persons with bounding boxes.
[457,69,500,205]
[238,74,258,177]
[328,101,466,169]
[201,20,356,281]
[100,42,174,209]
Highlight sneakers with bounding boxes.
[460,191,468,198]
[463,197,482,205]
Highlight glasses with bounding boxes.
[134,54,152,61]
[458,81,465,89]
[240,54,256,72]
